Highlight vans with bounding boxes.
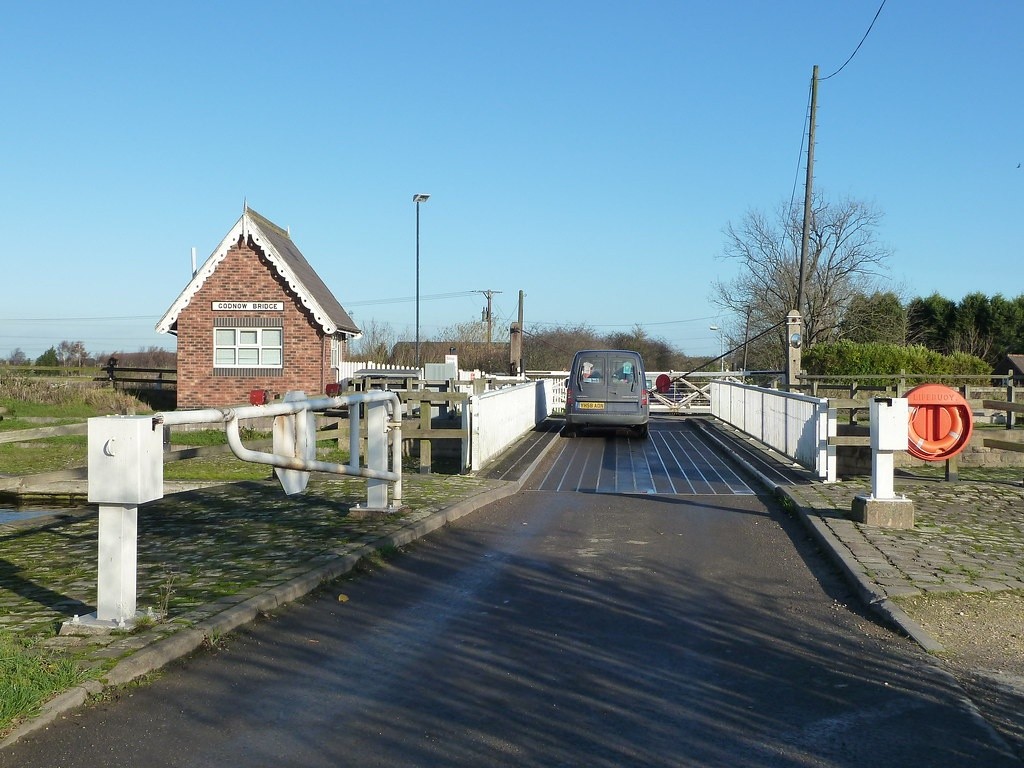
[565,351,652,440]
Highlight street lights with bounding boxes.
[518,292,527,372]
[413,193,431,369]
[711,326,724,373]
[449,346,456,354]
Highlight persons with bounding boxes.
[582,366,600,378]
[612,367,632,382]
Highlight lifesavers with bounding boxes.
[908,404,963,454]
